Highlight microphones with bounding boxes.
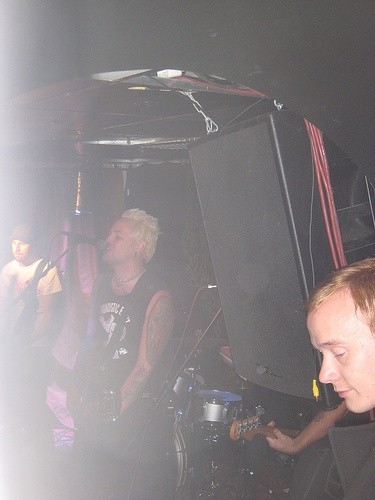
[74,168,83,218]
[61,231,105,250]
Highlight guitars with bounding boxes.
[228,404,328,443]
[66,369,119,426]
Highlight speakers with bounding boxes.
[329,421,375,500]
[188,111,375,399]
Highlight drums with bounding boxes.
[156,371,191,408]
[127,424,201,500]
[190,389,242,426]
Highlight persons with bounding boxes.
[75,208,174,500]
[263,397,370,500]
[0,224,62,430]
[306,257,375,413]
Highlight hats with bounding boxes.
[11,224,36,245]
